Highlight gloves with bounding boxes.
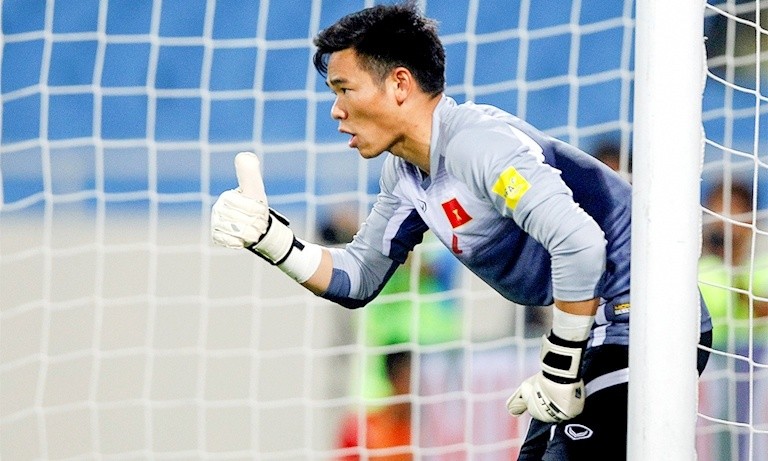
[211,151,306,266]
[504,329,586,424]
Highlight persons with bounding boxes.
[695,180,768,343]
[348,345,427,461]
[210,1,713,461]
